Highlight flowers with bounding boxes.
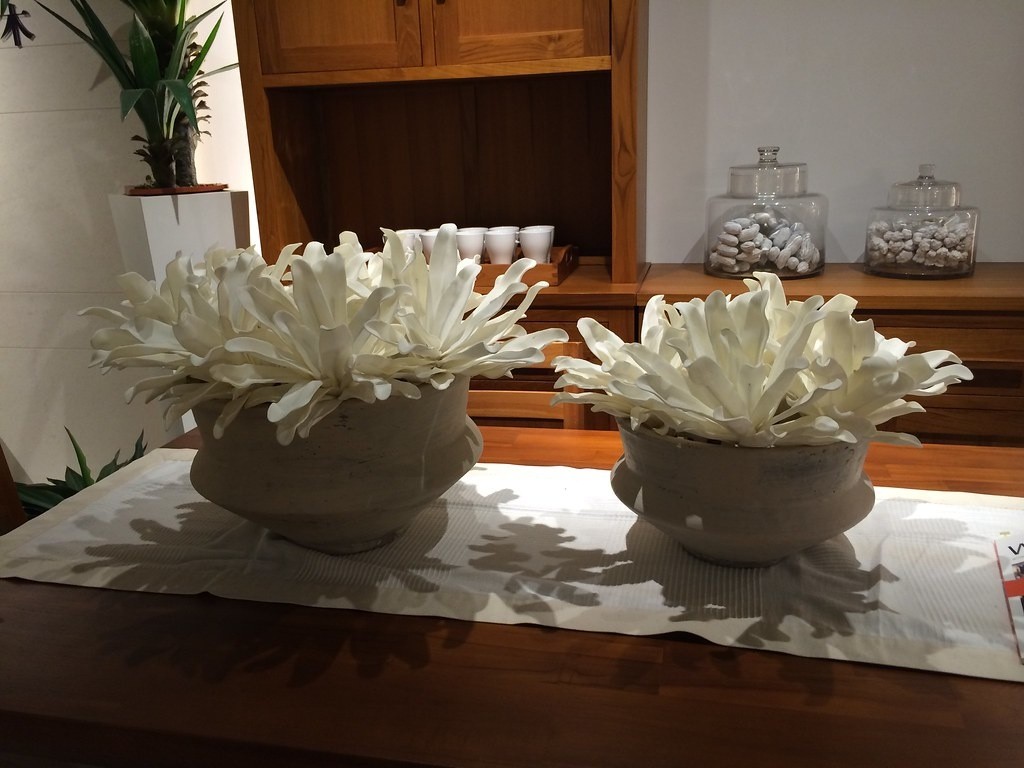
[76,224,569,446]
[551,271,976,446]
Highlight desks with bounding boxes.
[0,425,1023,768]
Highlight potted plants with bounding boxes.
[35,0,251,435]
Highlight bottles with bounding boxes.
[706,145,827,280]
[864,164,981,280]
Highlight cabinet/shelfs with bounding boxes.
[231,0,1024,448]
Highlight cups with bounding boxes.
[396,225,554,265]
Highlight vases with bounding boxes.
[611,414,877,567]
[177,369,484,556]
